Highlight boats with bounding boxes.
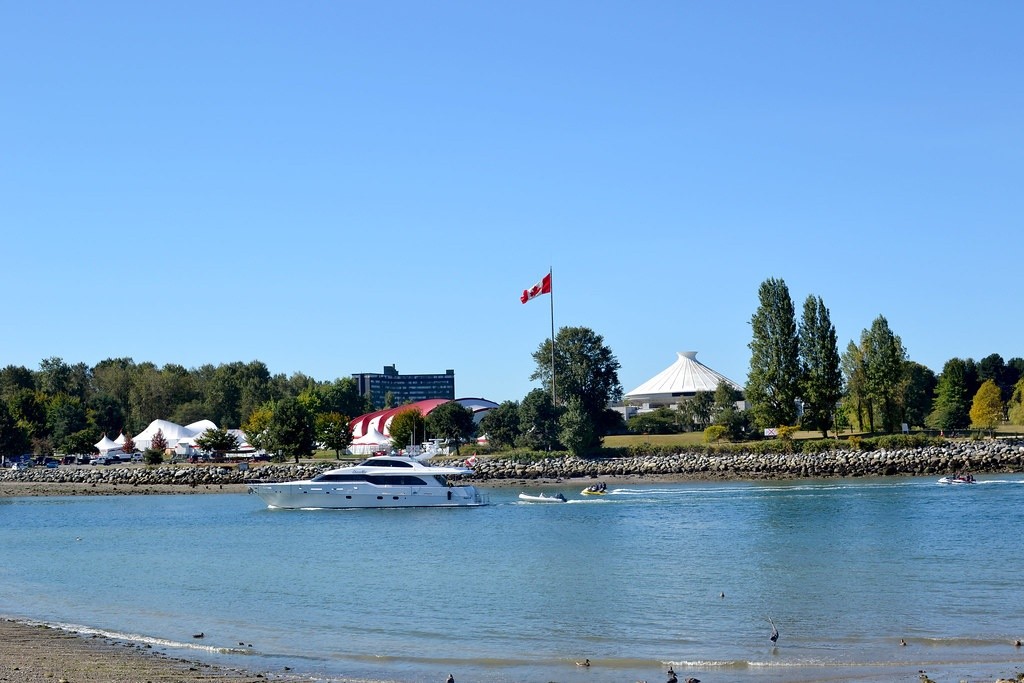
[939,478,967,486]
[583,487,608,495]
[247,453,491,508]
[519,493,566,503]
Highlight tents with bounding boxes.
[347,426,391,455]
[94,433,129,456]
[131,418,277,458]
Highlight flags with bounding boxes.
[466,453,475,467]
[521,272,551,304]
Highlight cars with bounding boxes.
[11,454,131,472]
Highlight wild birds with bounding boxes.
[192,591,906,683]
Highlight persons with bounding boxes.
[952,470,973,481]
[596,482,606,490]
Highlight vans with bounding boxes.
[133,453,143,462]
